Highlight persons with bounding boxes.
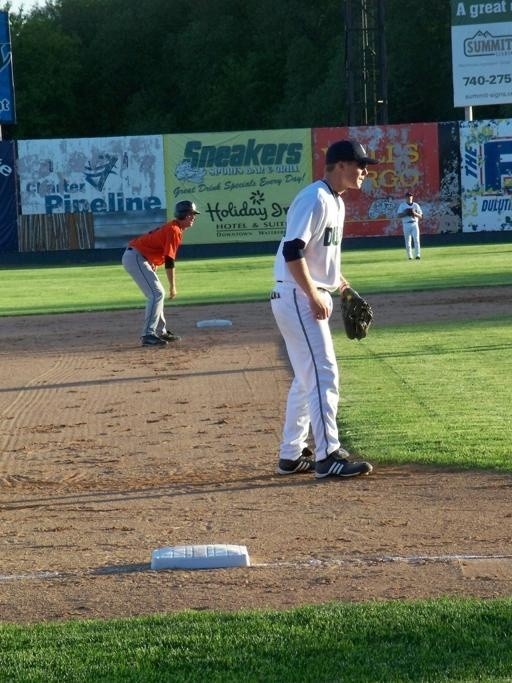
[270,137,381,482]
[395,191,423,259]
[121,199,202,347]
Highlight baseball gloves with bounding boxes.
[405,208,414,216]
[340,287,375,340]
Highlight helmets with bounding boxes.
[174,201,200,219]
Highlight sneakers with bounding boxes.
[315,447,373,478]
[416,257,420,259]
[159,332,180,342]
[278,448,316,474]
[141,334,167,346]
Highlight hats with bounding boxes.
[326,140,379,164]
[406,192,413,196]
